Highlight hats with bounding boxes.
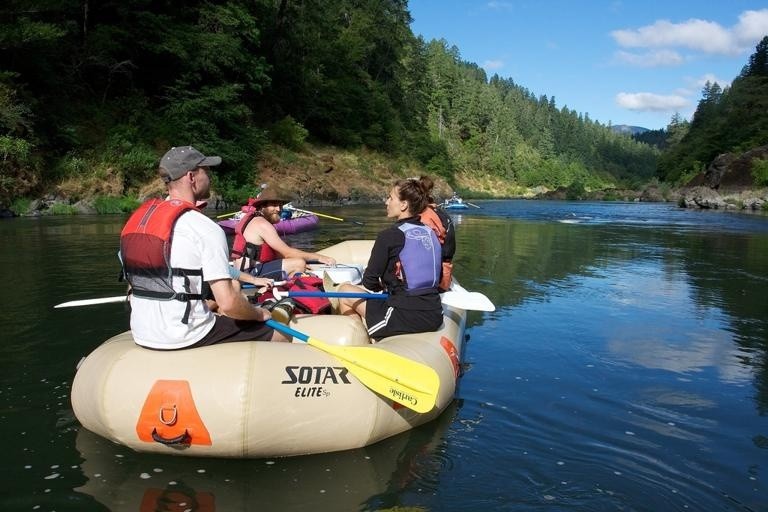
[159,146,222,183]
[251,185,294,208]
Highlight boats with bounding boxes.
[214,213,320,237]
[71,239,467,459]
[443,202,469,209]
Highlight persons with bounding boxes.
[241,198,256,213]
[257,184,267,198]
[428,196,456,293]
[230,186,336,284]
[118,146,292,351]
[281,202,292,220]
[450,192,463,203]
[338,175,444,344]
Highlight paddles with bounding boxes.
[264,319,440,413]
[54,296,129,309]
[466,203,484,212]
[273,287,496,313]
[291,209,365,227]
